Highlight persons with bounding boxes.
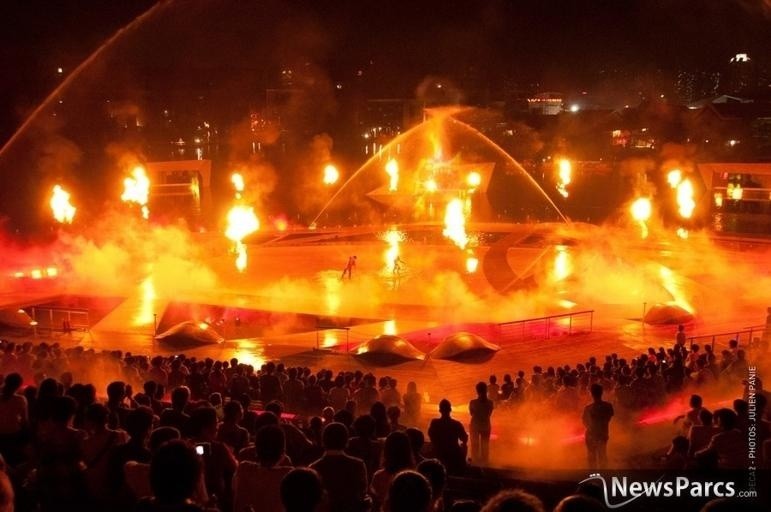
[765,307,771,335]
[0,335,770,512]
[675,325,688,348]
[340,254,358,282]
[392,255,407,277]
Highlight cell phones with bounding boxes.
[195,442,211,456]
